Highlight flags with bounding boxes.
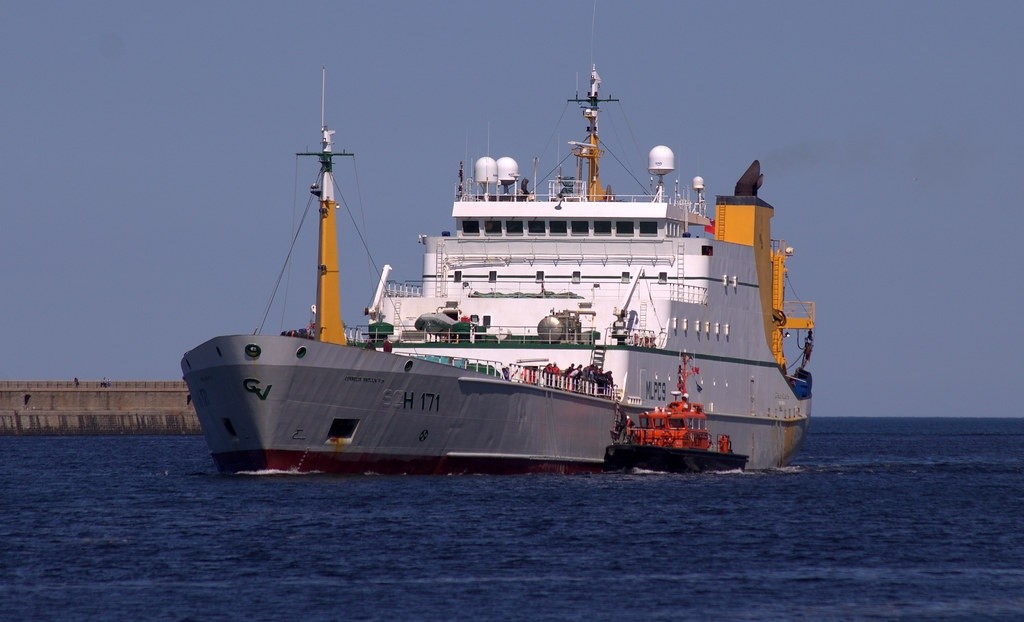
[703,216,717,236]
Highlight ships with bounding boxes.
[181,64,816,479]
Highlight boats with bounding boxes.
[602,347,750,475]
[413,312,455,337]
[453,321,486,341]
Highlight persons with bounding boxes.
[363,338,376,351]
[382,335,392,353]
[616,409,635,444]
[542,362,614,397]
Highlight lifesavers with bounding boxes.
[640,336,657,348]
[633,333,639,346]
